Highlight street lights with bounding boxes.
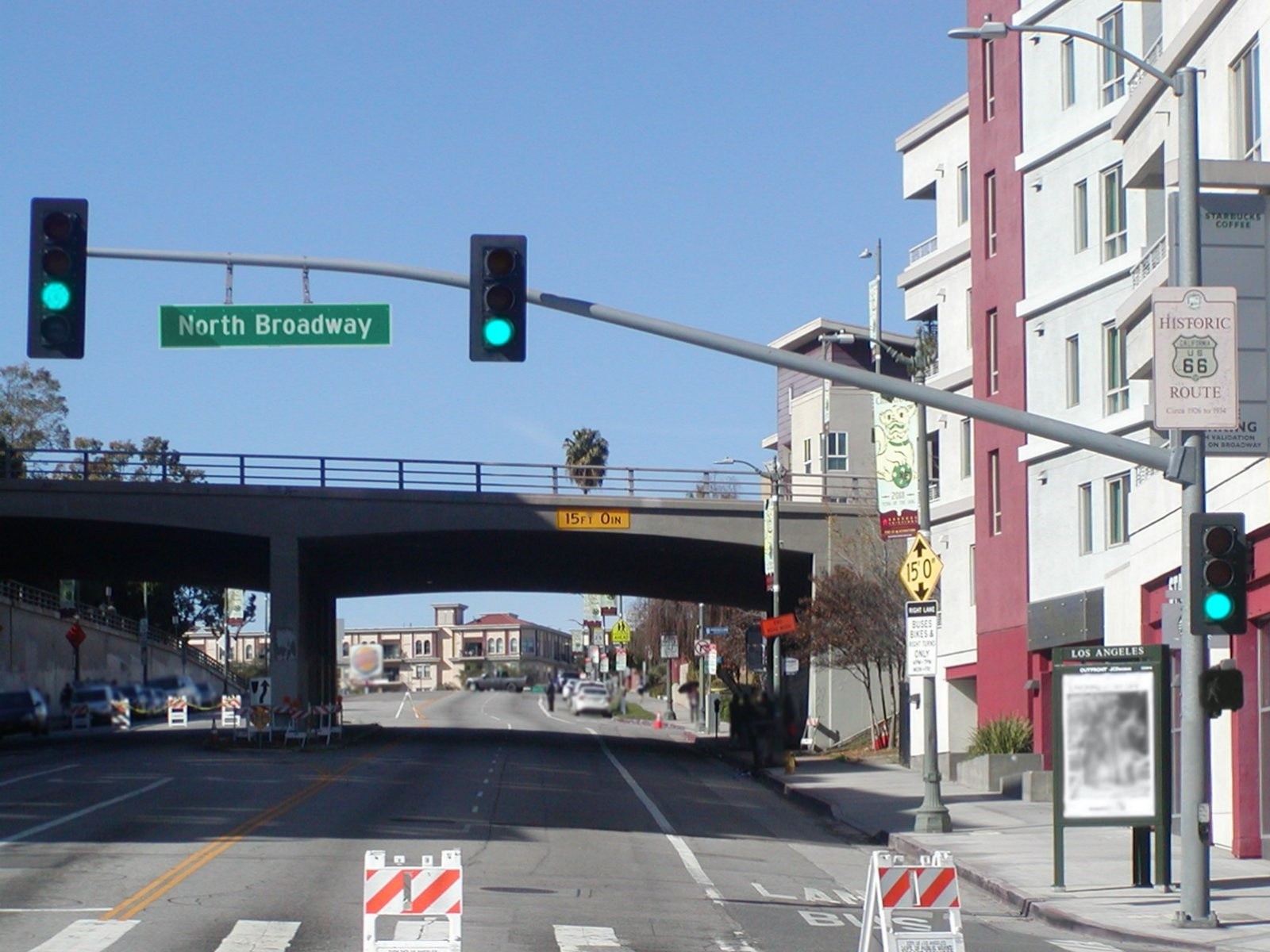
[858,236,882,378]
[817,331,951,833]
[946,20,1215,932]
[712,456,787,767]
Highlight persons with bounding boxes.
[33,679,117,717]
[546,679,556,712]
[636,682,644,702]
[689,685,700,723]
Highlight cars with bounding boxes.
[557,672,612,717]
[66,673,217,726]
[0,688,48,735]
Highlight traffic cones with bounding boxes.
[654,713,663,729]
[874,721,891,749]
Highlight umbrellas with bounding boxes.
[678,681,699,693]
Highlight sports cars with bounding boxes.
[466,673,529,694]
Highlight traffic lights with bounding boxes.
[28,197,90,359]
[1204,665,1243,711]
[1192,511,1246,638]
[469,233,526,361]
